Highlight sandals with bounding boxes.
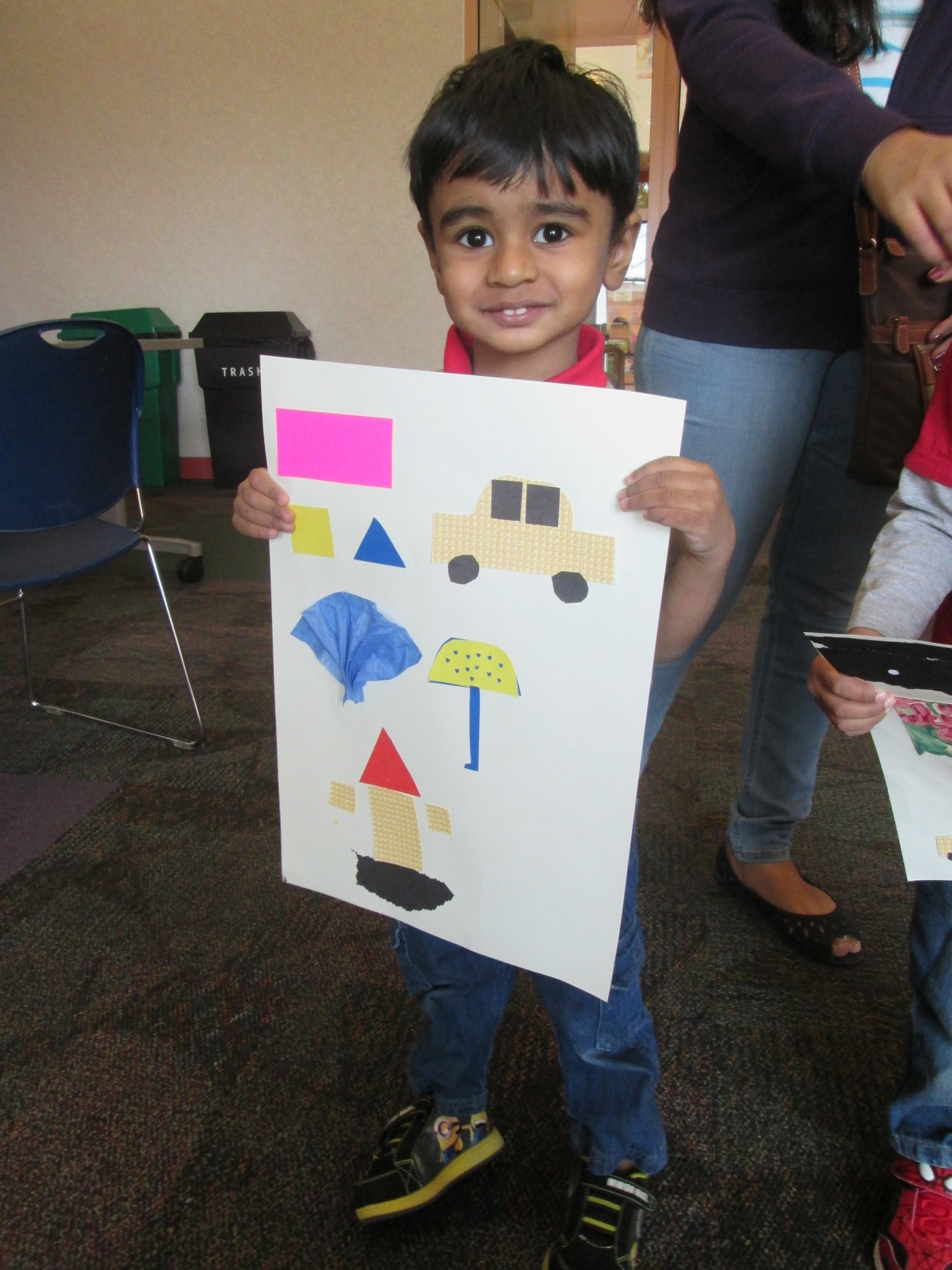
[714,839,866,967]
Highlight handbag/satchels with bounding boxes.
[851,228,951,488]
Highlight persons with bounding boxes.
[232,38,733,1269]
[811,350,952,1270]
[641,0,952,957]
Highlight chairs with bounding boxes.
[1,318,203,750]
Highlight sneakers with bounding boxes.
[542,1154,658,1270]
[355,1099,505,1222]
[873,1134,951,1269]
